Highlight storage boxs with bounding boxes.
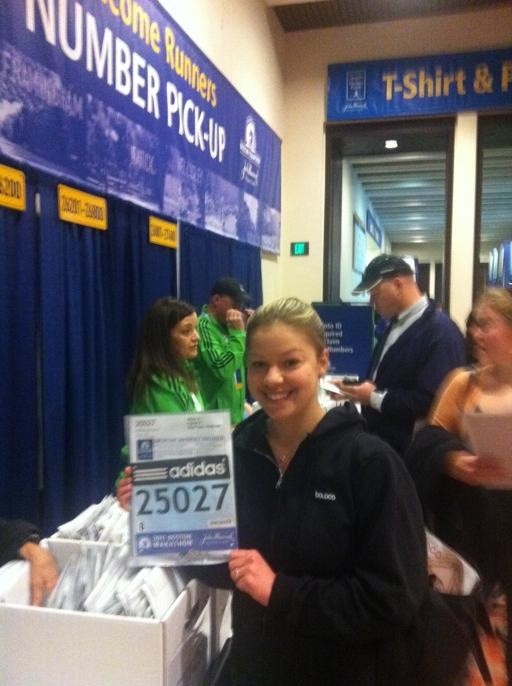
[0,538,212,685]
[48,495,131,546]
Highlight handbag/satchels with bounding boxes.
[402,535,484,685]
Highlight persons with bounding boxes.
[114,297,207,492]
[420,288,511,686]
[115,296,428,686]
[0,517,59,608]
[196,277,255,428]
[324,253,468,458]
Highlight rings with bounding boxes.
[234,567,240,580]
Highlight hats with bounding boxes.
[352,254,413,295]
[212,278,255,304]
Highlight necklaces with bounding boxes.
[267,439,300,464]
